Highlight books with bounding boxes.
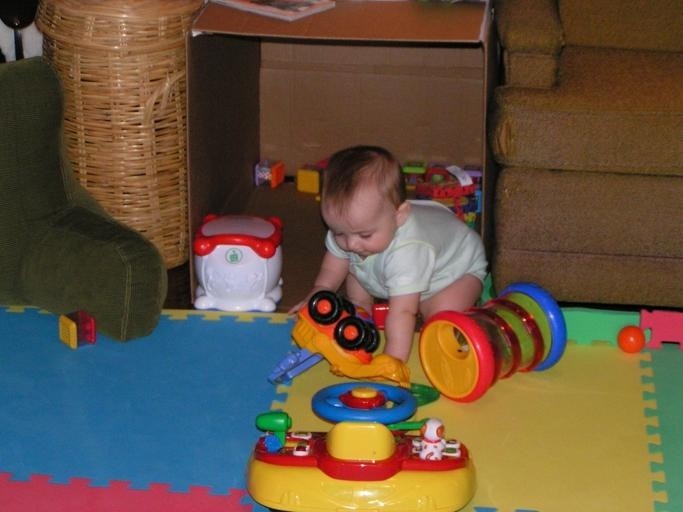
[216,0,336,22]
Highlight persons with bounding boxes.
[289,146,489,363]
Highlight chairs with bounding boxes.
[489,1,683,308]
